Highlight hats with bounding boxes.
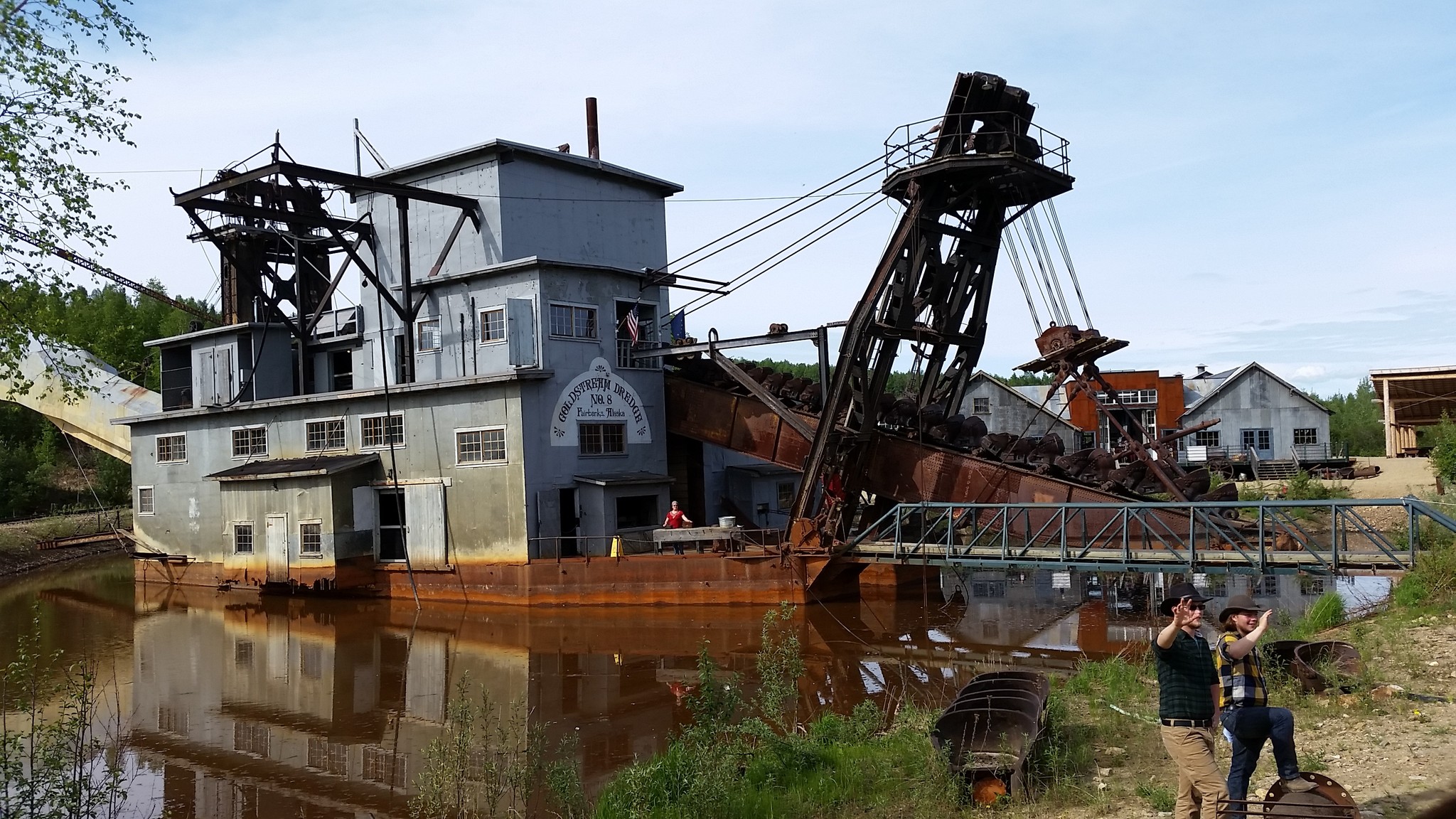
[1160,582,1214,617]
[1218,594,1269,622]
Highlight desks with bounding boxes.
[653,525,746,556]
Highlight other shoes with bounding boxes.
[676,553,679,555]
[681,553,684,554]
[1280,778,1319,793]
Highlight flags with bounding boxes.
[670,308,685,342]
[625,302,639,346]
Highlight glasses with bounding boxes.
[674,505,678,506]
[1184,604,1206,610]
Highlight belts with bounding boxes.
[1161,718,1214,727]
[1224,704,1242,710]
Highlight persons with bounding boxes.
[663,501,693,555]
[1214,594,1319,819]
[1150,581,1233,819]
